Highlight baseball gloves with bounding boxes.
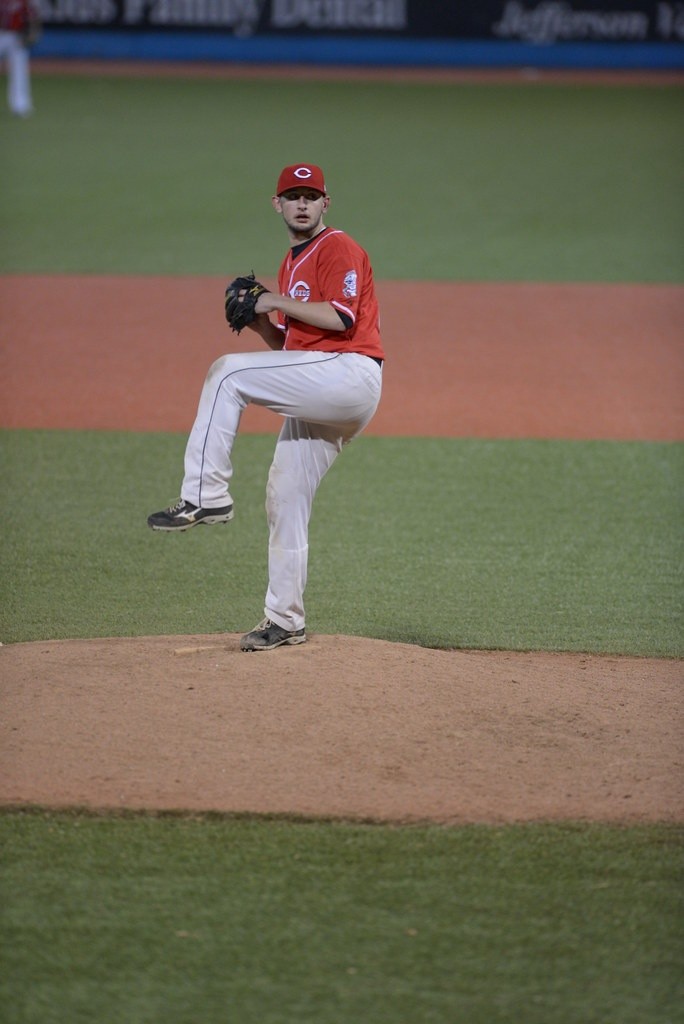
[224,273,272,336]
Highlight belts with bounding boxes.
[369,355,382,367]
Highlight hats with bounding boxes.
[276,164,326,197]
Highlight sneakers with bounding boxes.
[239,620,306,652]
[148,498,235,533]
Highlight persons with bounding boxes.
[0,0,37,114]
[148,163,385,652]
